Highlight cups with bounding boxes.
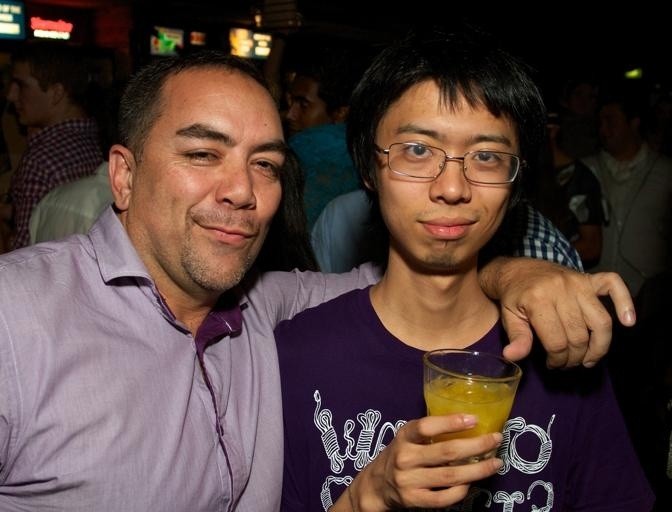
[422,347,522,470]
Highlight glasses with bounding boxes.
[372,140,524,184]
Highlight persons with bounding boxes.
[0,46,636,511]
[275,27,659,511]
[1,1,671,326]
